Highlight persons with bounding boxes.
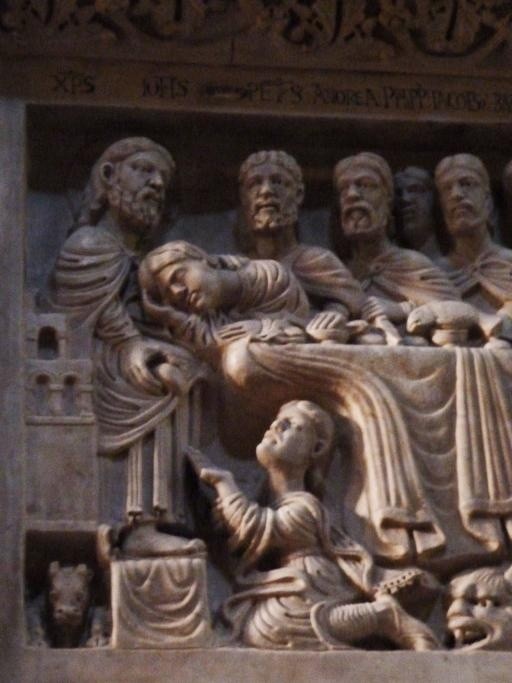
[394,163,442,259]
[42,134,179,400]
[401,150,511,350]
[136,235,313,348]
[183,393,434,654]
[238,147,365,343]
[336,145,470,346]
